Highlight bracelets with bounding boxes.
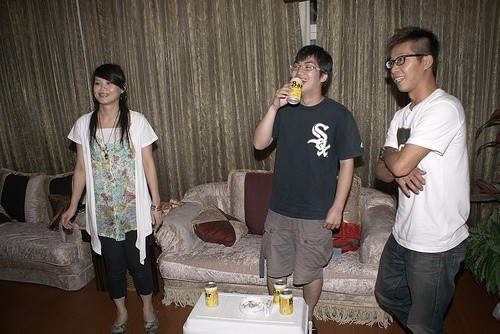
[151,205,162,211]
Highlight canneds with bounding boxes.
[286,77,303,104]
[273,280,286,304]
[279,288,293,315]
[205,281,219,307]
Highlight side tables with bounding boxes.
[81,230,159,294]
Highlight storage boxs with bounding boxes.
[183,292,312,334]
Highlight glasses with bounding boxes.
[384,53,428,69]
[289,62,321,72]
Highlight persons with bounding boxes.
[253,44,364,334]
[374,27,470,334]
[61,64,163,334]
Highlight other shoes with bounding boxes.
[142,311,159,334]
[111,315,129,334]
[308,321,318,334]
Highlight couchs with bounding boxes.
[156,182,396,330]
[0,168,96,292]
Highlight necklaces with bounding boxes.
[99,109,120,159]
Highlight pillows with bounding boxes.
[245,173,273,234]
[192,205,248,247]
